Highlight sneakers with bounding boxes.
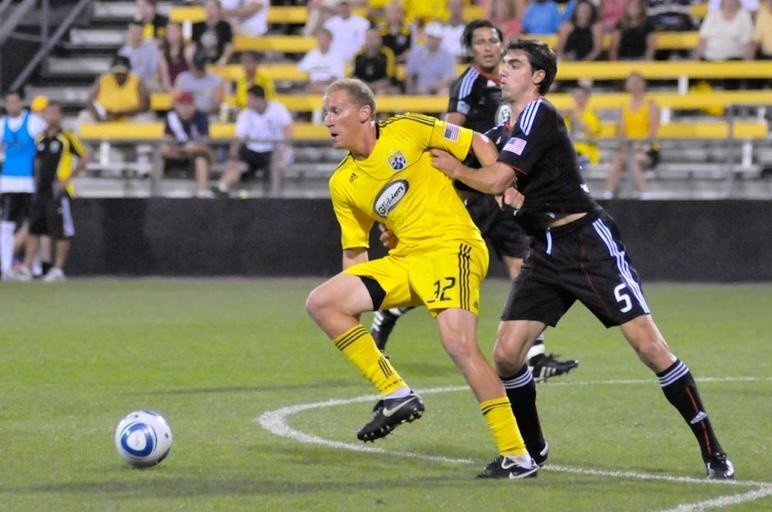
[532,441,550,464]
[357,388,426,442]
[475,456,539,479]
[532,353,579,383]
[1,260,65,282]
[705,453,734,480]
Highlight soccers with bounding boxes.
[116,410,172,467]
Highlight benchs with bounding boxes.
[76,4,772,179]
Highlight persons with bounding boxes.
[0,89,88,283]
[305,78,540,481]
[79,0,662,198]
[379,38,733,480]
[370,19,579,382]
[645,0,772,116]
